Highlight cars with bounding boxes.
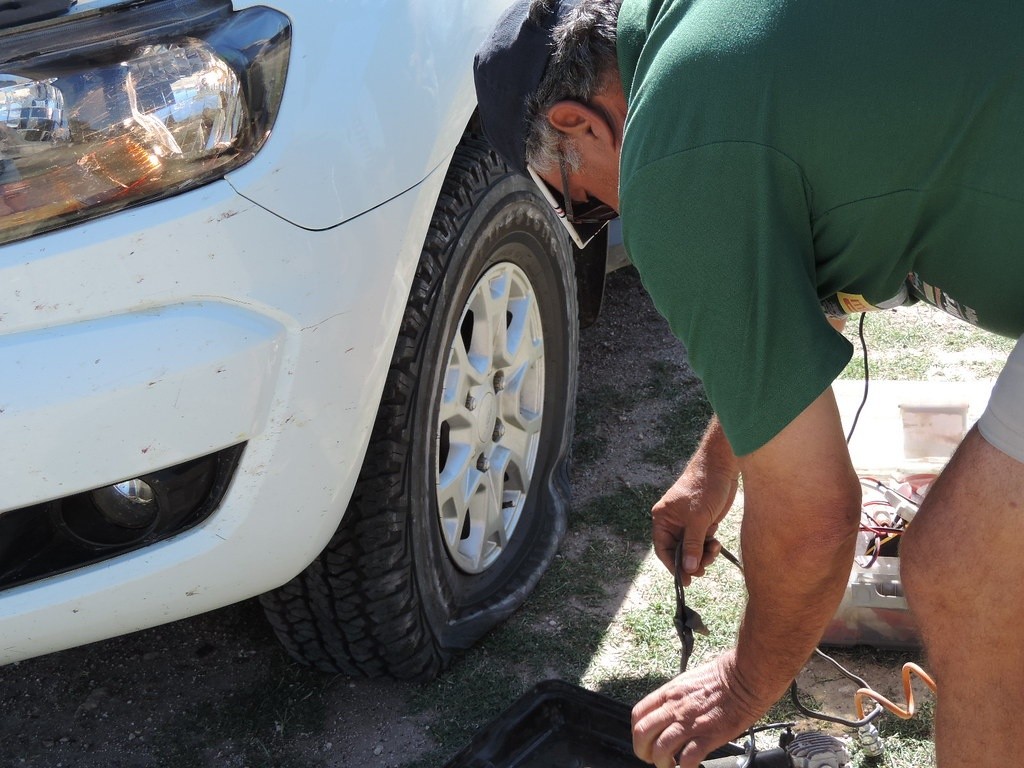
[0,1,629,661]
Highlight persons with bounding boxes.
[474,0,1024,768]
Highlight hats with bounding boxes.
[472,0,610,248]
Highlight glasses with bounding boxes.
[554,131,621,224]
